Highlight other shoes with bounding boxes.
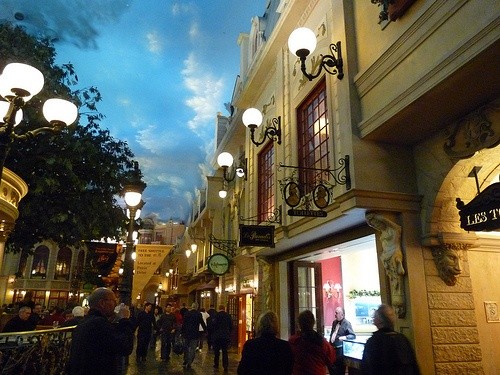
[183,360,188,365]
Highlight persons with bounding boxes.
[67,287,133,375]
[17,292,35,314]
[179,302,208,367]
[2,307,37,333]
[157,304,176,359]
[136,300,222,354]
[237,310,294,375]
[288,310,336,375]
[362,305,420,375]
[436,247,465,278]
[211,305,233,371]
[62,306,85,327]
[2,300,72,320]
[329,307,356,375]
[136,303,157,362]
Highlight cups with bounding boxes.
[53,321,58,329]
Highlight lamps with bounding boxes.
[206,291,211,296]
[284,28,347,84]
[253,281,257,290]
[191,234,204,253]
[168,267,174,275]
[185,243,192,259]
[214,286,221,294]
[236,155,246,177]
[218,184,228,198]
[216,151,248,183]
[165,271,170,278]
[200,290,205,297]
[240,107,282,148]
